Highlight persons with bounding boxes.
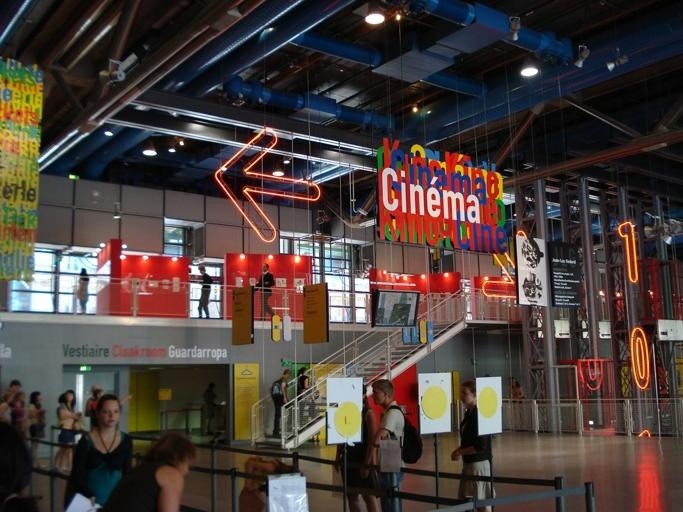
[0,378,219,512]
[255,262,276,322]
[334,383,382,511]
[363,380,408,512]
[236,451,295,512]
[75,269,90,314]
[449,380,497,512]
[272,369,295,438]
[297,366,316,431]
[510,377,525,404]
[197,265,214,318]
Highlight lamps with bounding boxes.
[507,16,629,79]
[98,53,138,84]
[364,0,389,25]
[642,206,682,246]
[142,136,158,157]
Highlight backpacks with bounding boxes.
[386,406,421,464]
